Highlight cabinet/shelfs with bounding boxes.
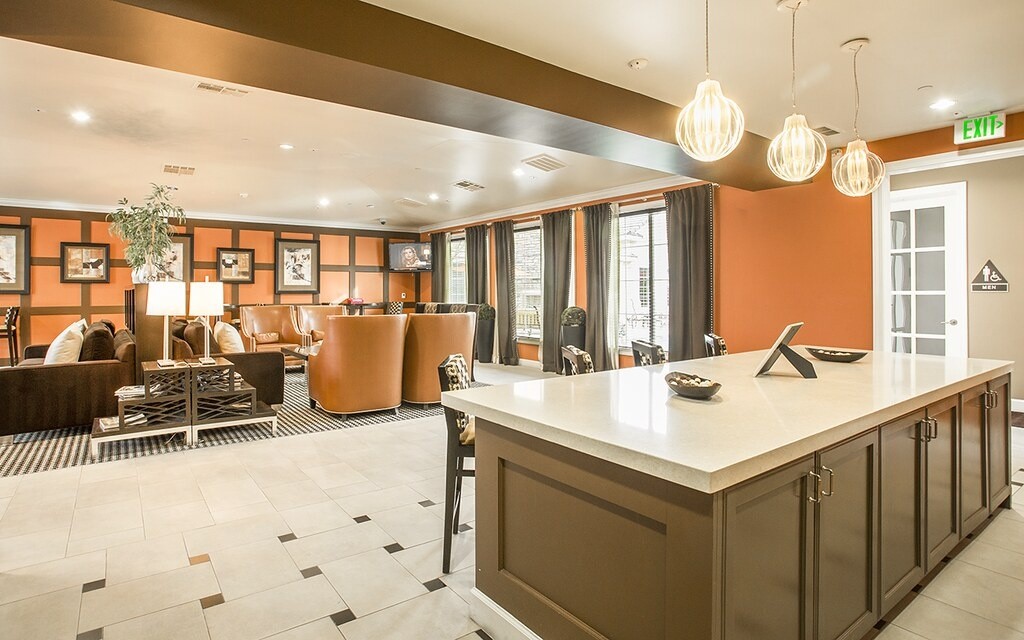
[90,357,278,460]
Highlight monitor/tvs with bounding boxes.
[388,242,432,271]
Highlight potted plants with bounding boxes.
[560,306,585,349]
[477,303,495,363]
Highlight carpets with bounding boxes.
[0,361,488,478]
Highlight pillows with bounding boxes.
[214,321,246,352]
[77,318,88,333]
[43,322,83,366]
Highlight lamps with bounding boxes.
[145,276,185,367]
[188,276,224,364]
[674,0,745,162]
[831,38,885,196]
[767,0,827,183]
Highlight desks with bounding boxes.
[339,303,386,315]
[440,345,1015,640]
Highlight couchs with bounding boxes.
[307,314,408,420]
[239,304,307,369]
[296,306,346,345]
[0,328,135,436]
[402,312,476,410]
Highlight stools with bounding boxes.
[0,307,20,366]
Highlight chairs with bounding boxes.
[561,345,594,376]
[631,339,665,366]
[703,333,727,357]
[438,354,475,573]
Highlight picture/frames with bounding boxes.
[59,242,110,283]
[0,224,30,294]
[167,233,194,282]
[754,321,804,378]
[274,238,320,294]
[216,248,255,284]
